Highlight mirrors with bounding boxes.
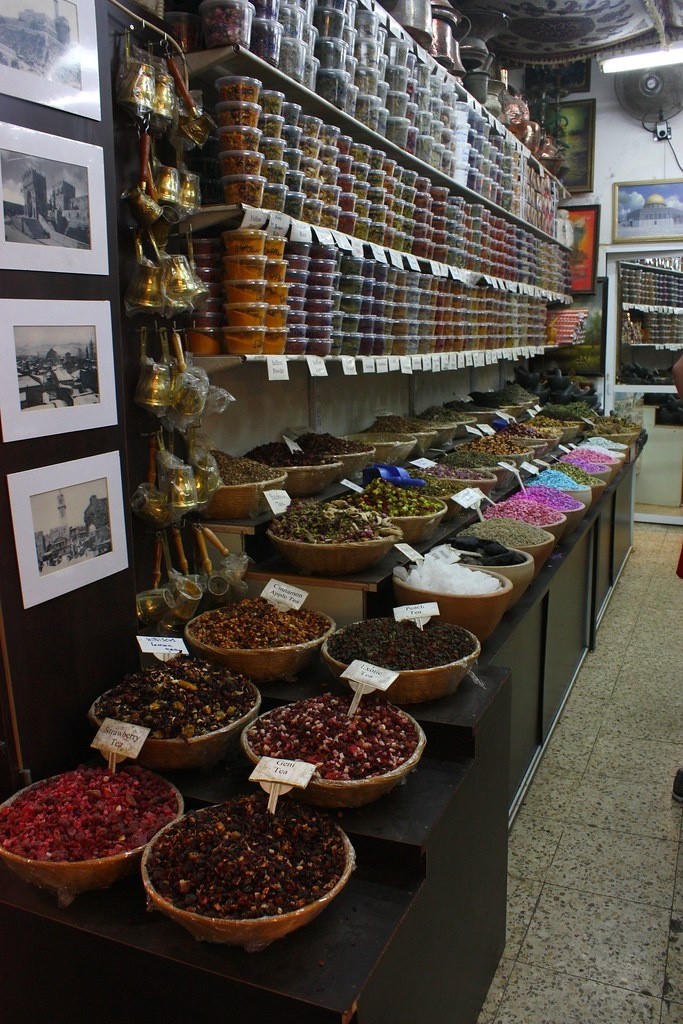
[603,249,683,525]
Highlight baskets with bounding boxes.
[201,394,644,576]
[87,682,262,773]
[319,617,481,704]
[0,766,185,909]
[141,797,356,953]
[239,698,428,808]
[184,601,337,690]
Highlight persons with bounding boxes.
[673,356,683,579]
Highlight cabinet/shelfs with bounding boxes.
[612,263,683,508]
[0,1,683,1024]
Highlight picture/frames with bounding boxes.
[525,55,592,94]
[556,204,601,296]
[529,98,596,193]
[4,450,129,610]
[0,120,111,277]
[0,297,119,443]
[0,0,102,122]
[612,178,683,244]
[555,276,608,376]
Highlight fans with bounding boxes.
[614,63,683,140]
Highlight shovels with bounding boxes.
[492,418,533,432]
[362,464,426,488]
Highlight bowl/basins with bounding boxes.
[622,256,683,343]
[144,2,572,356]
